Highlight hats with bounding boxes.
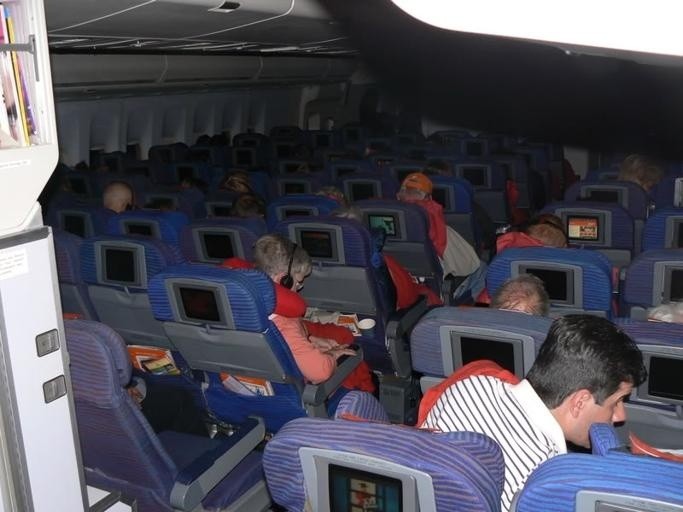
[403,173,433,193]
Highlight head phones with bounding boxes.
[281,236,297,288]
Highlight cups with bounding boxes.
[359,319,376,337]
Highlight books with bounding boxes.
[0,0,39,148]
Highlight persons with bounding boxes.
[25,115,683,451]
[411,313,648,512]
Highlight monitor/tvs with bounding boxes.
[100,247,137,284]
[521,264,573,304]
[664,266,683,301]
[175,283,223,326]
[316,457,415,512]
[566,213,604,242]
[638,351,682,404]
[451,331,521,376]
[586,188,620,205]
